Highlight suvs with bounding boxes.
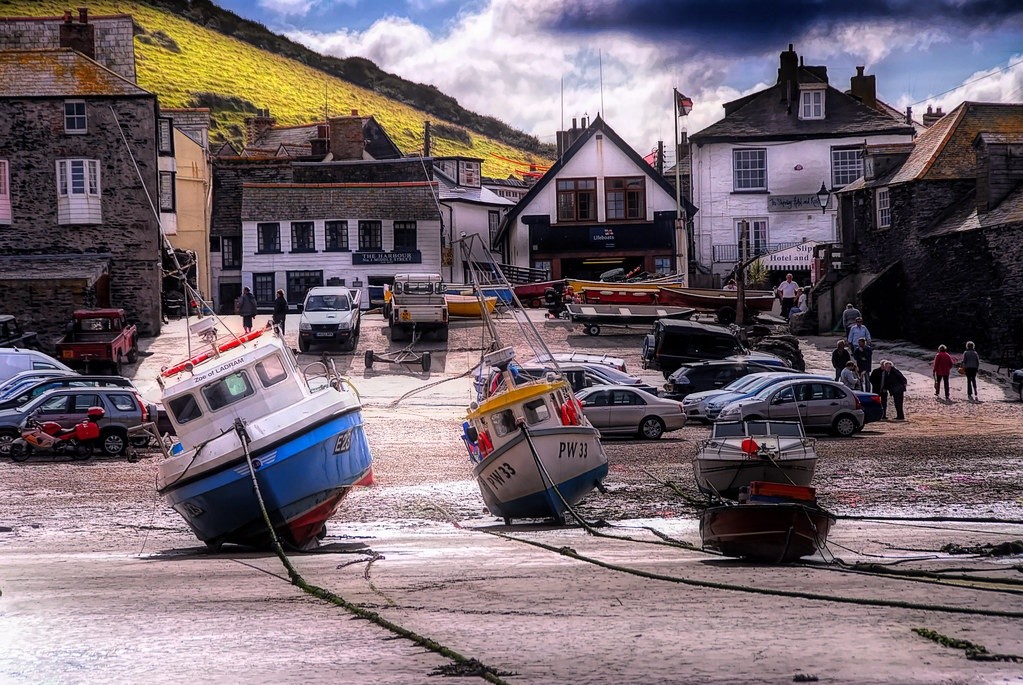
[661,354,778,401]
[640,319,793,381]
[0,387,158,458]
[0,370,142,448]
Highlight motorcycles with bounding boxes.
[9,406,106,463]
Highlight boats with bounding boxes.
[445,278,775,330]
[108,103,375,555]
[419,152,609,523]
[692,379,836,564]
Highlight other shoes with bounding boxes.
[968,395,973,400]
[934,393,938,396]
[894,417,904,420]
[946,396,949,399]
[975,396,978,401]
[882,417,888,419]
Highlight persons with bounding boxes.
[237,287,256,333]
[832,340,850,381]
[933,345,952,400]
[843,304,862,340]
[840,361,861,388]
[272,289,288,335]
[961,341,979,402]
[787,288,808,322]
[773,286,780,298]
[723,279,737,290]
[778,273,800,318]
[884,361,907,420]
[848,317,871,352]
[854,338,872,391]
[870,360,889,419]
[563,286,575,301]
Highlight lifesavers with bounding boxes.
[561,399,583,428]
[478,431,493,458]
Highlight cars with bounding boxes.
[1011,369,1023,404]
[535,386,687,441]
[474,351,666,405]
[0,314,38,350]
[682,353,885,433]
[718,377,865,438]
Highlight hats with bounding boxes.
[855,317,863,322]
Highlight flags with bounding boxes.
[677,92,693,117]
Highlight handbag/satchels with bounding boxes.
[958,364,965,374]
[933,375,938,389]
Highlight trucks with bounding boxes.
[390,271,450,343]
[55,308,137,376]
[296,286,361,353]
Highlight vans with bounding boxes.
[0,348,76,390]
